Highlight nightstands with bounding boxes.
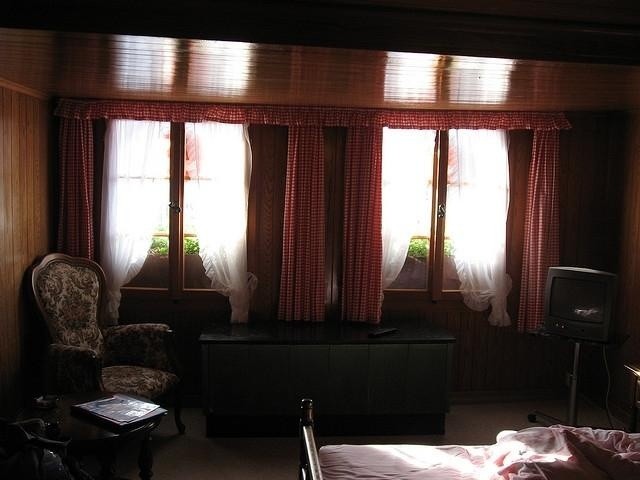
[625,360,640,434]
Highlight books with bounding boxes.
[71,394,168,429]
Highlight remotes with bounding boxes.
[370,326,397,337]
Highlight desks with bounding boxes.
[528,331,630,426]
[195,325,455,436]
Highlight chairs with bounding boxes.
[20,252,191,439]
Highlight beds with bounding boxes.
[296,397,640,480]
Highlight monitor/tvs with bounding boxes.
[543,267,618,344]
